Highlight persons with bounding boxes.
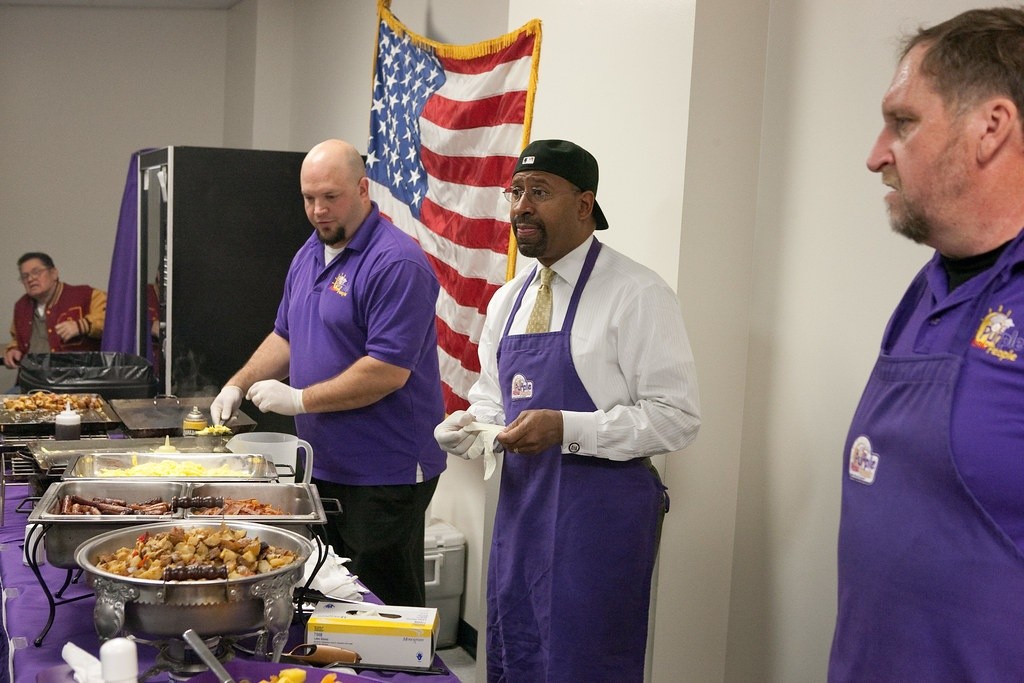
[434,139,701,683]
[828,8,1024,683]
[147,264,164,378]
[2,252,109,395]
[210,139,448,607]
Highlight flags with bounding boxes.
[365,0,544,414]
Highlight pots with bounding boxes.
[73,497,314,637]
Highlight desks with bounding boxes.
[0,428,460,683]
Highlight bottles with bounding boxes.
[55,400,81,441]
[154,435,180,455]
[182,406,208,437]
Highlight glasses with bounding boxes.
[502,186,582,204]
[19,267,49,282]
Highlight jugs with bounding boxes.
[225,432,314,485]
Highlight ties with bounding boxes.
[525,267,557,334]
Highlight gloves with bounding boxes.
[463,421,506,481]
[209,385,243,428]
[245,379,306,416]
[433,410,484,461]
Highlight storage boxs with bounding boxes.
[424,521,466,649]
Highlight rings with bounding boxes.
[514,448,519,454]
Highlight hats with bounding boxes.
[512,140,609,230]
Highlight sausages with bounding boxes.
[61,494,171,515]
